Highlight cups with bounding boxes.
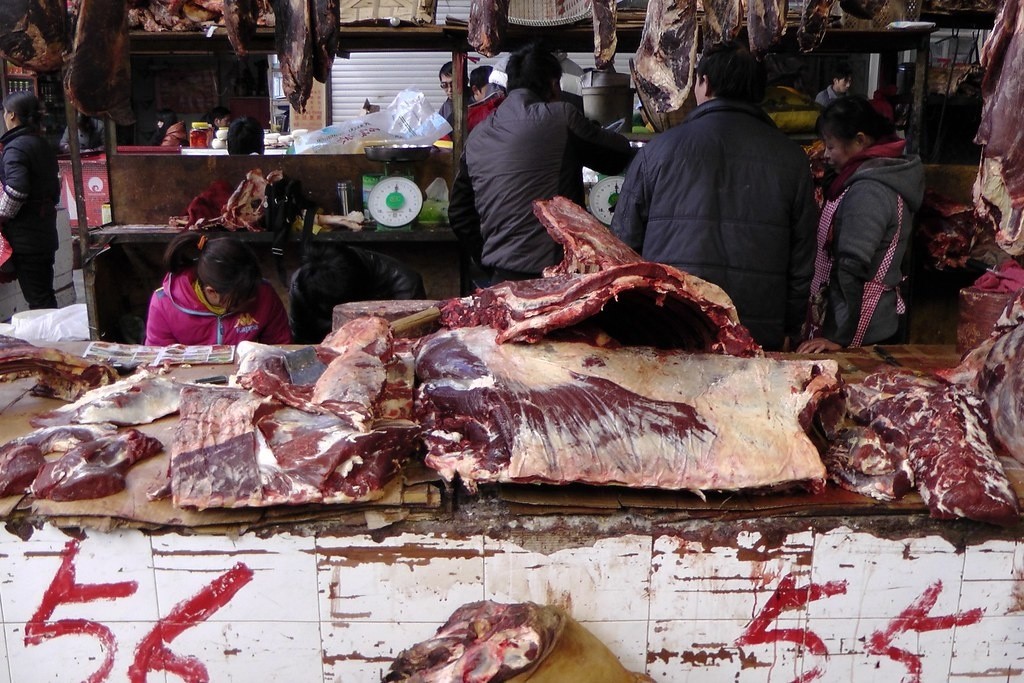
[271,114,287,133]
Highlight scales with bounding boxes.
[589,173,628,226]
[363,141,432,231]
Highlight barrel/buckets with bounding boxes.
[581,86,637,133]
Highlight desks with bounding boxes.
[1,336,1024,683]
[59,144,189,230]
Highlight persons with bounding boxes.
[287,242,428,345]
[143,231,292,346]
[60,108,106,156]
[610,41,823,359]
[0,91,59,310]
[815,66,856,107]
[437,46,598,143]
[147,109,188,147]
[797,94,925,353]
[210,106,231,129]
[227,117,268,155]
[445,45,631,294]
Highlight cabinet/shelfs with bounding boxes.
[59,0,939,345]
[0,56,66,137]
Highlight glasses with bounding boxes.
[440,81,453,89]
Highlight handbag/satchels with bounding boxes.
[259,177,302,234]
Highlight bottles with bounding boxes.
[40,81,57,103]
[207,124,214,148]
[189,122,211,149]
[7,80,32,95]
[337,180,355,216]
